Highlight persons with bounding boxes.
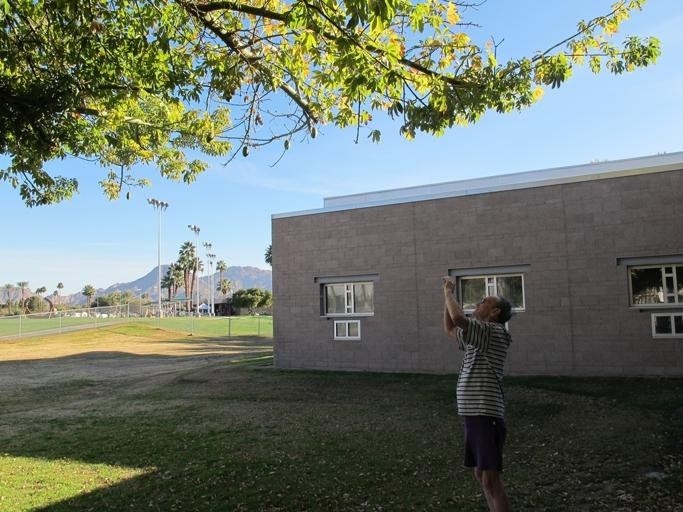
[441,276,512,512]
